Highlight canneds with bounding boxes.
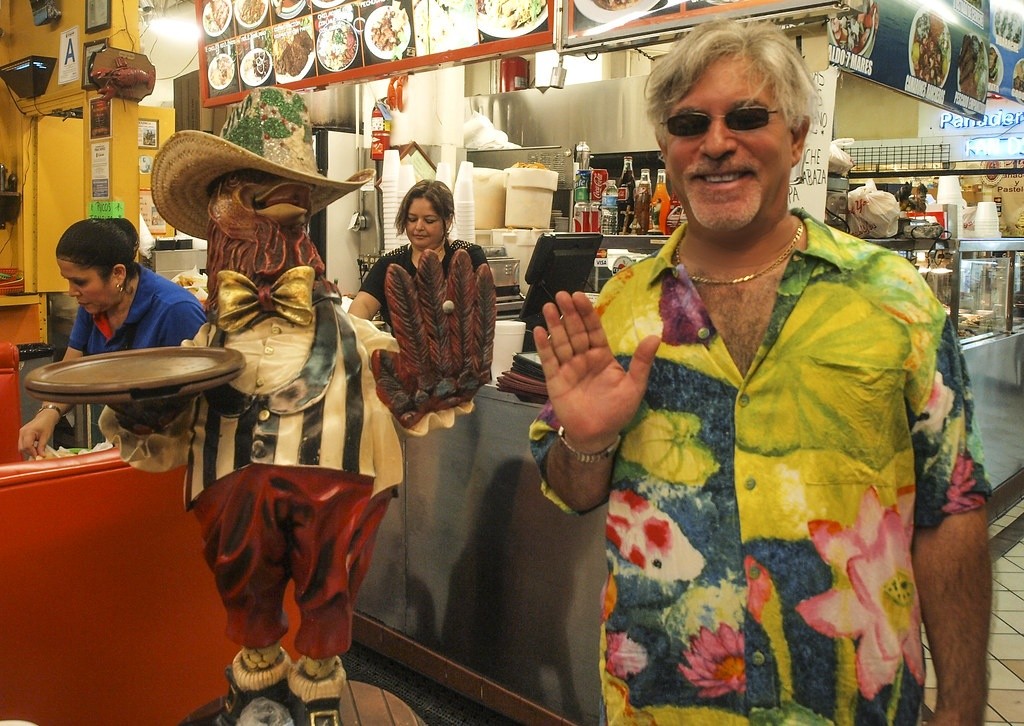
[573,168,608,232]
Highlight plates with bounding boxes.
[473,0,661,38]
[202,1,411,89]
[829,3,1024,117]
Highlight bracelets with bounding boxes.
[557,426,621,463]
[39,405,61,415]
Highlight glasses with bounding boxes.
[660,106,778,137]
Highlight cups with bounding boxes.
[490,320,525,381]
[382,149,476,256]
[933,176,999,239]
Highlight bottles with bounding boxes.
[601,157,671,234]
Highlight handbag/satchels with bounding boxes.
[829,138,856,174]
[846,179,901,239]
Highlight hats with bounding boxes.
[150,86,377,238]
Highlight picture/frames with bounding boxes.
[81,37,109,88]
[85,0,112,33]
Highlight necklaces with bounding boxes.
[675,221,804,285]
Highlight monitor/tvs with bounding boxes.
[518,232,605,318]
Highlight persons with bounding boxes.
[528,20,994,726]
[348,180,488,338]
[18,219,206,460]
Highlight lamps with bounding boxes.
[0,55,57,99]
